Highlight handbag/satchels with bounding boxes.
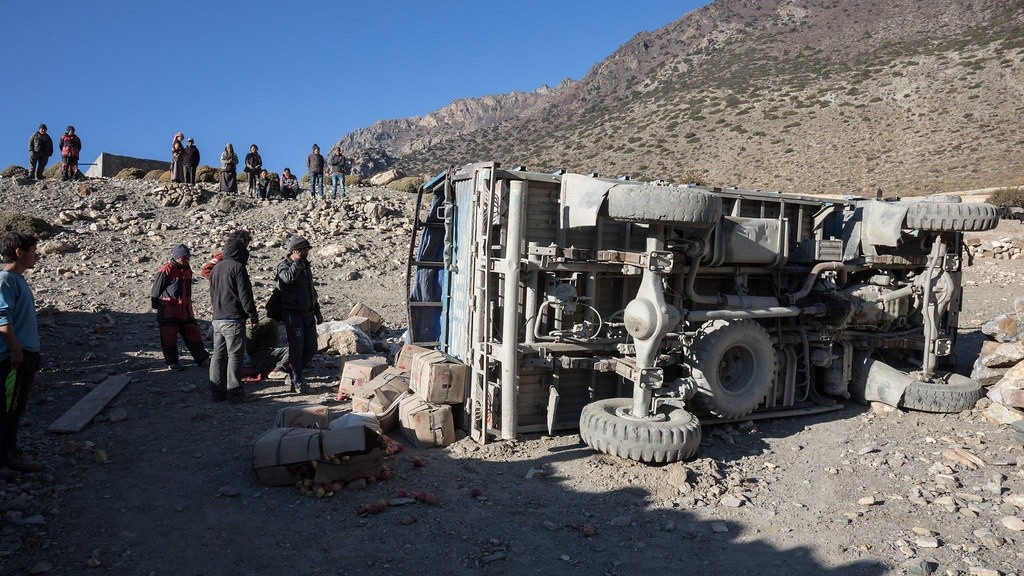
[265,288,284,322]
[339,166,348,173]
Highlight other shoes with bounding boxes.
[240,367,261,382]
[290,381,316,394]
[301,366,312,374]
[168,363,185,371]
[214,390,239,401]
[0,467,23,479]
[9,453,44,472]
[201,356,211,366]
[237,394,259,403]
[267,371,289,379]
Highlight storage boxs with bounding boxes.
[251,344,466,486]
[348,302,381,333]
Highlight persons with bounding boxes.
[209,238,259,405]
[151,244,212,372]
[28,124,53,180]
[170,132,185,183]
[256,169,272,201]
[307,144,325,198]
[219,143,238,193]
[0,231,43,481]
[274,237,323,393]
[330,147,346,200]
[59,126,81,181]
[201,230,253,280]
[184,138,200,185]
[280,168,299,202]
[245,144,262,198]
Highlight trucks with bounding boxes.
[399,160,1003,461]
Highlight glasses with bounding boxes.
[179,256,190,262]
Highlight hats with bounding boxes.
[66,126,75,131]
[173,244,191,261]
[312,144,319,151]
[187,138,194,144]
[289,234,313,254]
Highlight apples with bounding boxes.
[289,435,481,525]
[583,524,593,537]
